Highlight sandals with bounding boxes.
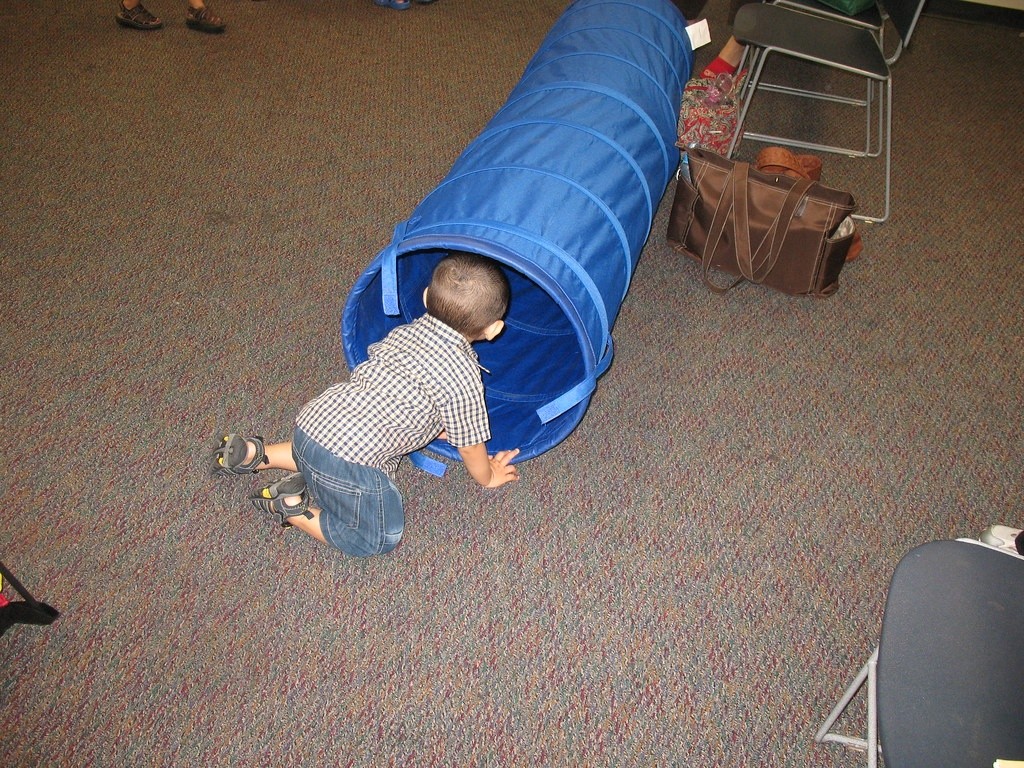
[247,472,313,530]
[116,0,162,29]
[187,5,228,32]
[209,434,265,477]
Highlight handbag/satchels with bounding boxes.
[679,69,746,158]
[666,145,861,303]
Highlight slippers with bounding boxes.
[700,57,742,79]
[374,0,434,11]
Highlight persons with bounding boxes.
[374,0,439,10]
[115,0,226,30]
[671,0,770,80]
[210,251,519,558]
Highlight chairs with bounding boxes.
[814,539,1024,768]
[719,0,924,222]
